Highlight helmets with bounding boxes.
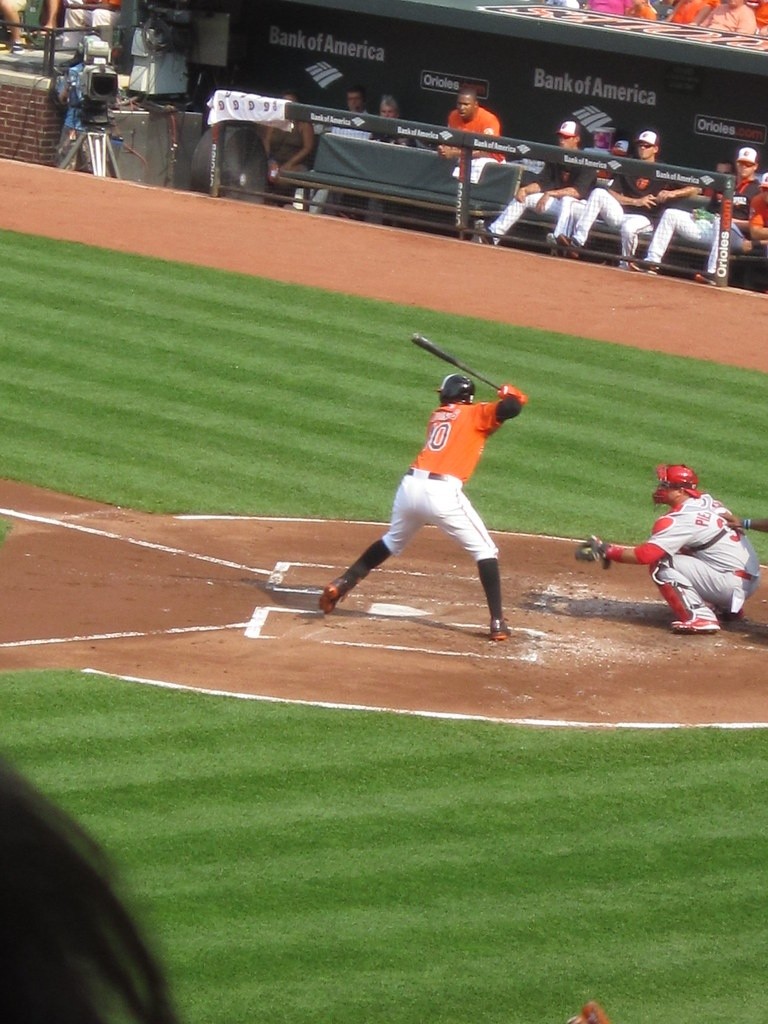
[661,466,700,499]
[436,373,475,403]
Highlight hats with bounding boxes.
[737,146,759,165]
[759,172,768,188]
[636,131,658,146]
[556,121,580,136]
[613,141,631,155]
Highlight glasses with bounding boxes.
[762,187,768,192]
[559,134,576,139]
[639,144,655,149]
[738,161,754,167]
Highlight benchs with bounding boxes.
[515,178,768,263]
[275,132,526,244]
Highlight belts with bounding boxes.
[406,469,442,480]
[733,571,752,580]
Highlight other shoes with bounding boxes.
[696,273,716,285]
[62,58,80,67]
[628,259,657,276]
[11,41,24,55]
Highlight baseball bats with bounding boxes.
[410,332,500,391]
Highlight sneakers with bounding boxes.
[476,219,493,247]
[546,233,558,259]
[672,617,715,634]
[557,233,580,259]
[708,604,738,621]
[319,576,349,614]
[490,617,511,641]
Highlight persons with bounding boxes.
[260,87,401,226]
[575,464,760,634]
[750,171,768,250]
[475,121,597,245]
[559,130,661,269]
[628,146,759,287]
[719,512,768,534]
[438,87,506,173]
[56,35,120,175]
[316,372,526,641]
[0,0,123,50]
[542,0,768,39]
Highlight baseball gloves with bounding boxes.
[575,534,610,570]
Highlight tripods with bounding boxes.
[58,124,122,180]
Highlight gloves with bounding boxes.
[497,384,528,405]
